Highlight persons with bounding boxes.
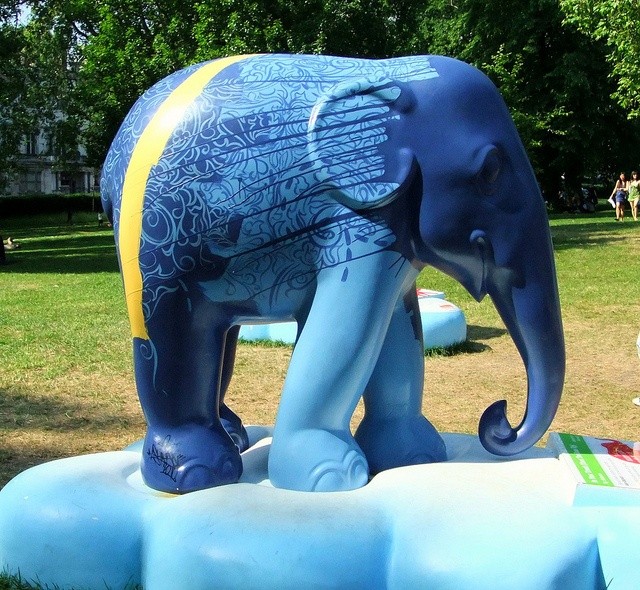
[609,171,629,222]
[98,212,104,228]
[628,170,640,221]
